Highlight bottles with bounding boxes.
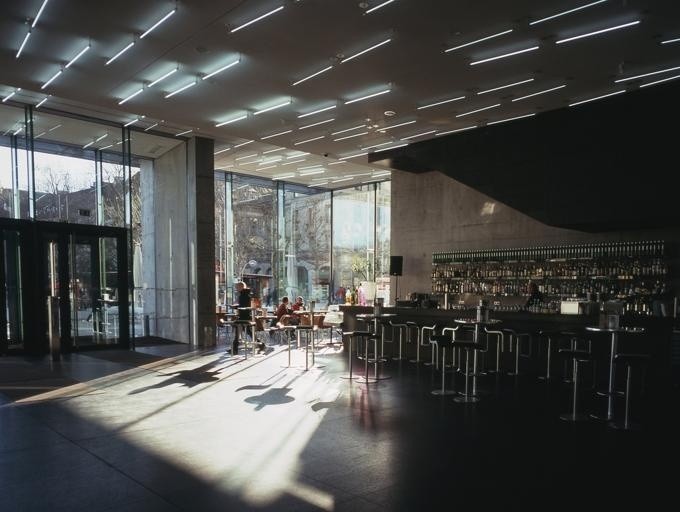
[511,248,514,260]
[523,248,526,259]
[529,247,532,260]
[661,240,664,255]
[612,242,615,256]
[597,243,601,257]
[503,249,506,260]
[627,241,630,256]
[562,246,564,259]
[546,246,550,259]
[580,245,584,257]
[495,248,498,260]
[565,246,568,258]
[615,243,618,256]
[584,244,587,257]
[646,241,649,255]
[653,241,657,255]
[541,247,543,259]
[638,240,641,255]
[574,245,577,257]
[604,243,607,256]
[571,245,574,258]
[493,249,495,260]
[559,246,561,258]
[635,242,638,255]
[536,299,541,313]
[498,249,501,260]
[432,259,667,295]
[649,241,653,255]
[514,247,517,260]
[501,249,503,260]
[619,242,623,256]
[346,287,351,305]
[538,247,540,260]
[552,246,555,258]
[556,245,558,258]
[526,248,529,260]
[591,244,594,257]
[509,248,511,260]
[608,242,611,256]
[630,241,634,255]
[587,243,591,257]
[657,240,661,255]
[532,247,534,260]
[577,244,580,257]
[533,299,536,313]
[549,246,552,259]
[622,241,626,256]
[520,248,523,259]
[535,247,538,259]
[518,248,520,260]
[568,245,571,258]
[543,246,546,259]
[594,244,597,257]
[601,243,604,257]
[432,249,493,262]
[507,249,509,260]
[641,241,645,254]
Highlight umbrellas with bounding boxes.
[285,242,299,299]
[133,244,145,307]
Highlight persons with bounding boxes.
[291,296,307,321]
[522,284,546,308]
[358,283,367,305]
[336,287,345,304]
[225,282,267,354]
[346,288,350,297]
[270,297,296,340]
[349,290,359,307]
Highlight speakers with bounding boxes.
[389,255,403,276]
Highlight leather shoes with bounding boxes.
[226,349,238,355]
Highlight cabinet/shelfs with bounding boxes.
[429,252,669,311]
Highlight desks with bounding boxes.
[584,326,647,423]
[355,313,399,380]
[292,310,330,369]
[452,318,503,406]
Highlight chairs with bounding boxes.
[539,328,556,382]
[232,318,256,359]
[428,334,454,397]
[406,318,422,363]
[377,319,395,362]
[440,323,462,369]
[482,322,505,374]
[464,326,490,378]
[615,352,648,429]
[559,329,586,386]
[389,317,409,361]
[502,326,533,377]
[559,347,594,421]
[342,330,382,384]
[282,325,297,367]
[323,312,344,344]
[298,324,314,371]
[420,320,438,367]
[357,317,376,361]
